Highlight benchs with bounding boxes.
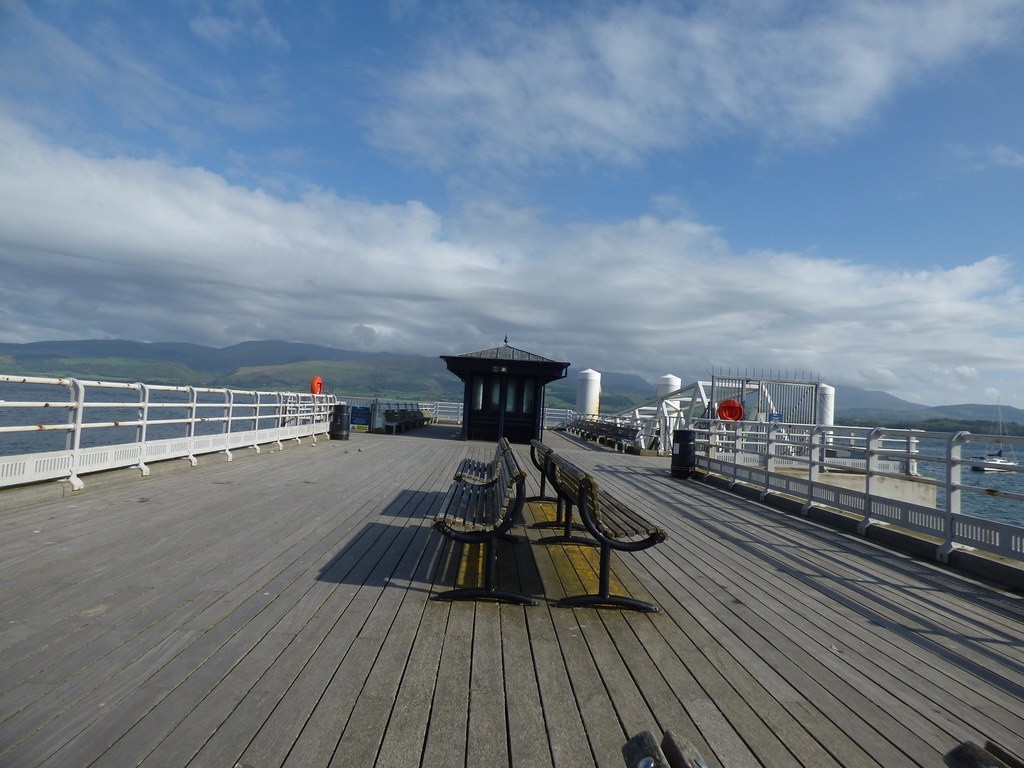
[453,437,513,483]
[524,439,587,531]
[554,453,669,613]
[429,447,539,606]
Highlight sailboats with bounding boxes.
[969,395,1020,471]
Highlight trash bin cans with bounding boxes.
[329,406,351,441]
[671,429,696,478]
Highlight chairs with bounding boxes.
[564,416,645,453]
[383,403,437,434]
